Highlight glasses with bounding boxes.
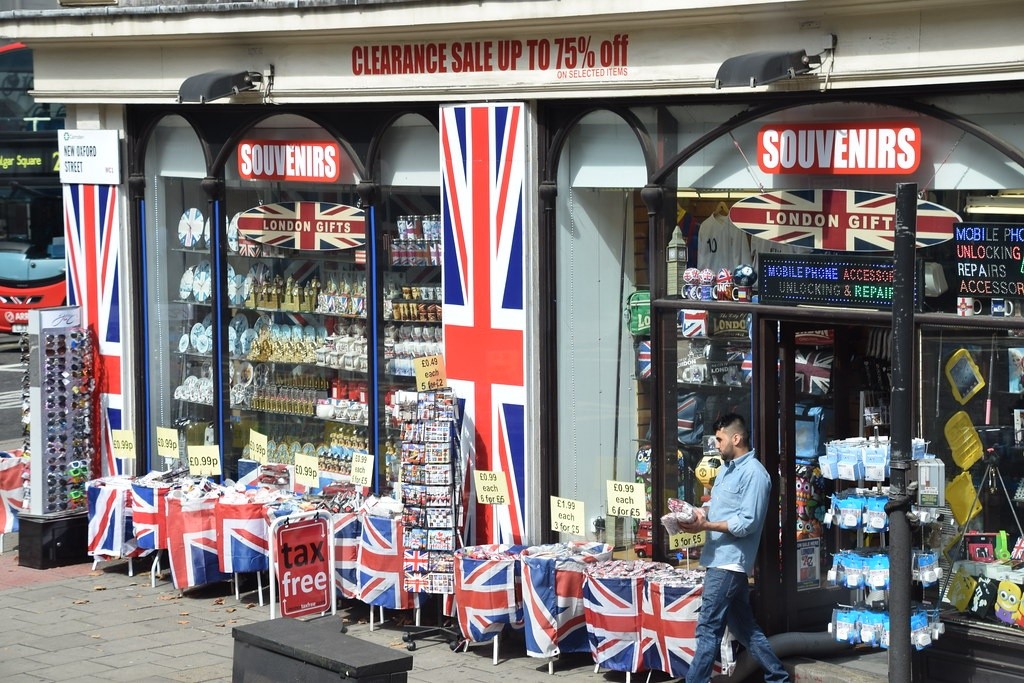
[18,332,31,510]
[45,330,95,512]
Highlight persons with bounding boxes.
[678,414,789,683]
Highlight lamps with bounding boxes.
[713,32,840,89]
[177,61,276,105]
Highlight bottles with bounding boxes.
[187,416,210,446]
[394,215,442,266]
[251,275,321,311]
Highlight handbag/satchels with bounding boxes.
[742,346,781,385]
[634,338,651,378]
[626,289,650,336]
[676,340,711,382]
[775,400,828,464]
[645,392,704,445]
[694,394,749,445]
[748,314,780,340]
[795,330,832,345]
[711,341,746,386]
[794,348,835,396]
[677,308,709,338]
[711,311,748,337]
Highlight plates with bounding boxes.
[174,312,327,404]
[178,207,270,304]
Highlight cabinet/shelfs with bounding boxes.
[158,188,446,494]
[21,303,93,519]
[632,295,889,487]
[819,434,945,650]
[383,385,468,650]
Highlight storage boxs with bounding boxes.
[0,446,33,555]
[81,474,737,683]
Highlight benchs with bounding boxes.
[230,615,414,682]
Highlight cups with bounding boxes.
[393,282,442,320]
[334,400,363,421]
[317,320,443,375]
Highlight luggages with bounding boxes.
[776,466,826,545]
[636,445,693,515]
